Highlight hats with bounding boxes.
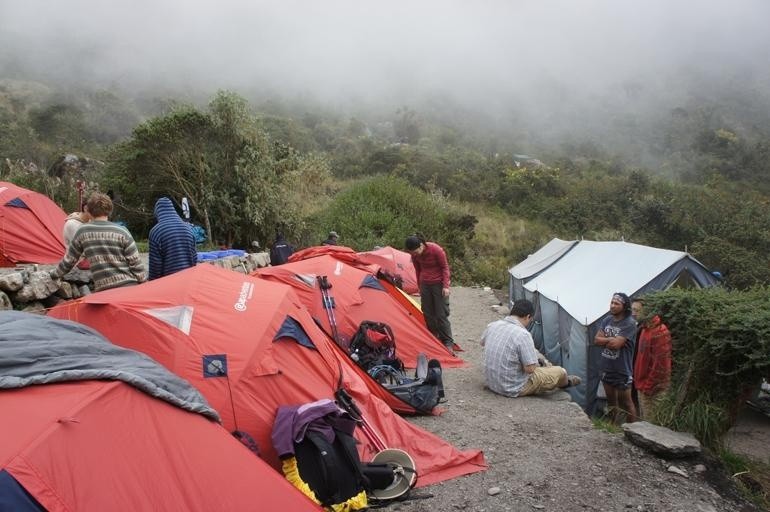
[363,447,416,500]
[329,230,340,238]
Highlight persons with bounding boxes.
[148,197,197,281]
[50,192,147,291]
[62,200,92,283]
[480,298,581,398]
[405,233,452,353]
[631,299,672,425]
[593,293,637,420]
[321,231,340,246]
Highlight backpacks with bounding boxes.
[291,409,366,507]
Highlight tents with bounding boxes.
[0,309,325,512]
[42,262,486,501]
[508,237,579,313]
[250,254,462,370]
[522,239,725,409]
[1,179,73,268]
[351,245,420,295]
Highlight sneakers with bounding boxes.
[560,375,583,389]
